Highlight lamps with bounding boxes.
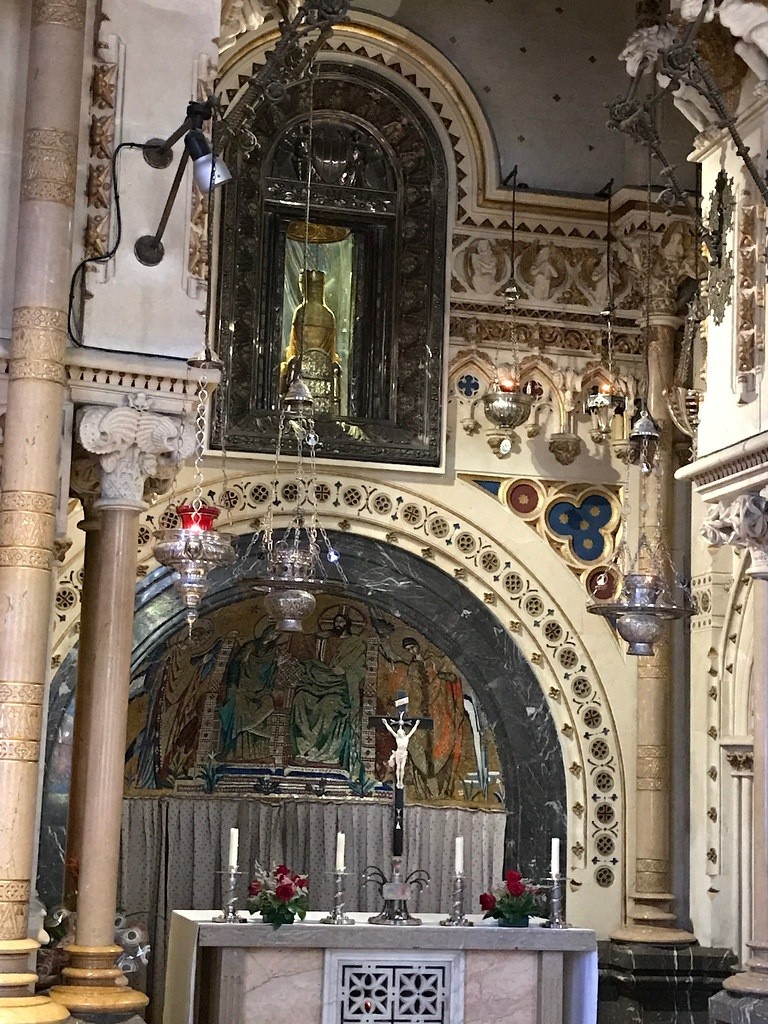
[151,77,238,642]
[587,178,626,439]
[481,163,533,455]
[588,139,698,658]
[134,102,231,267]
[232,61,348,634]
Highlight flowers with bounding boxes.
[479,868,544,924]
[245,860,310,930]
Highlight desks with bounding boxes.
[162,909,598,1024]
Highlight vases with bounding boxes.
[498,915,528,927]
[263,913,294,924]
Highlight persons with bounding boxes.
[382,717,420,791]
[472,241,500,293]
[593,251,617,309]
[529,245,560,301]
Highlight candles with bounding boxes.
[336,831,345,868]
[455,837,463,871]
[551,838,559,876]
[229,828,239,866]
